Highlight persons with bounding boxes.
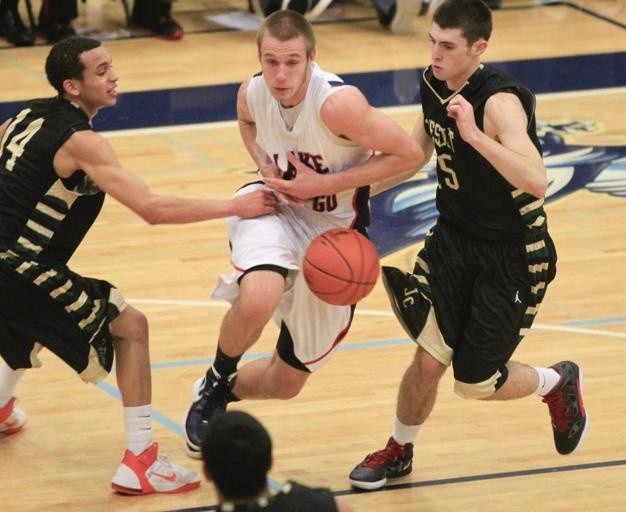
[131,0,185,41]
[1,36,279,498]
[347,2,585,494]
[2,0,79,48]
[183,7,426,461]
[183,410,339,511]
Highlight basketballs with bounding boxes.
[304,227,380,305]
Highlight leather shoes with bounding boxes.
[1,5,37,48]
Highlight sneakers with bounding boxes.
[184,364,241,457]
[33,16,79,44]
[126,13,187,41]
[110,443,202,496]
[346,437,416,492]
[0,396,27,436]
[545,360,587,457]
